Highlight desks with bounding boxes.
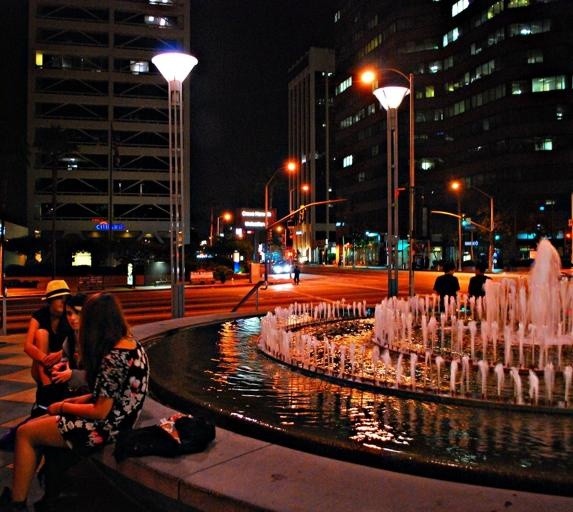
[275,225,286,247]
[289,183,310,215]
[358,64,420,300]
[447,179,466,273]
[152,50,200,319]
[262,156,299,285]
[216,212,233,237]
[286,249,292,279]
[371,83,409,300]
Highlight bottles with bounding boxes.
[42,280,71,300]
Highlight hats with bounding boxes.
[270,261,295,275]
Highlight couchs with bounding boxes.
[493,232,502,245]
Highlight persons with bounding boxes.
[468,262,491,298]
[434,262,460,312]
[1,278,151,511]
[293,265,300,285]
